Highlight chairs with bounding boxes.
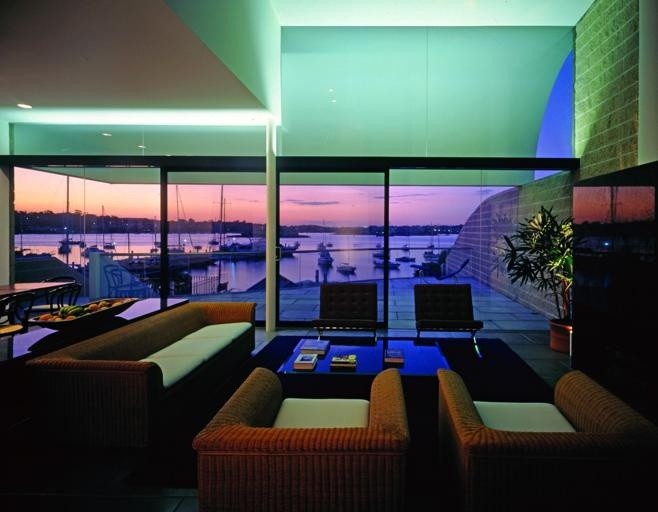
[312,282,486,362]
[0,274,82,331]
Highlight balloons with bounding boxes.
[300,339,330,355]
[293,353,318,370]
[330,354,357,368]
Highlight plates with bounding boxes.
[29,298,139,331]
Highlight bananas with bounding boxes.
[60,305,83,318]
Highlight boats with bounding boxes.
[317,240,356,275]
[372,242,440,271]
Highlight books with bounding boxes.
[384,348,404,364]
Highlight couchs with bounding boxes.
[23,300,258,477]
[438,367,655,511]
[192,367,409,511]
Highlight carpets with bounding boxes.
[180,335,555,442]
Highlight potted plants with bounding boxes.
[500,203,589,353]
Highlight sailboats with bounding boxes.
[58,176,301,279]
